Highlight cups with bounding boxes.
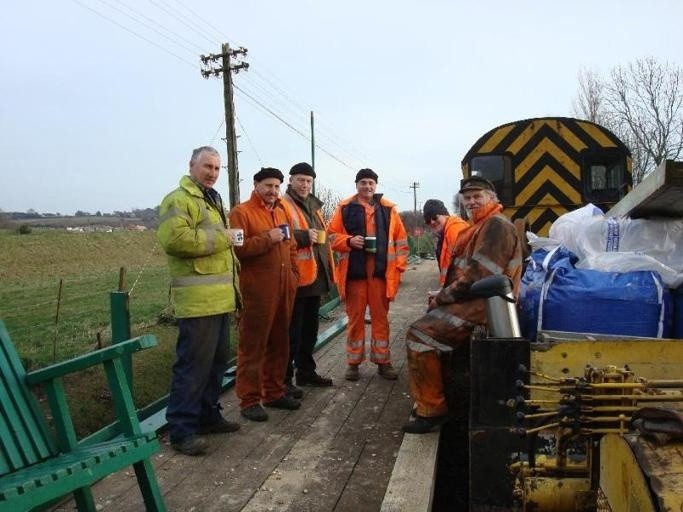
[277,224,291,241]
[363,236,376,252]
[228,228,244,248]
[313,230,326,245]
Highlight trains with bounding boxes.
[461,116,681,511]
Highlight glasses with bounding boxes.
[423,214,439,225]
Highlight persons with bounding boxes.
[157,146,244,456]
[228,167,301,423]
[424,198,471,313]
[402,176,523,433]
[282,161,333,399]
[327,169,410,381]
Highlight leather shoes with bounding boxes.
[295,372,332,386]
[262,396,300,410]
[399,415,449,434]
[376,363,397,380]
[212,418,240,432]
[344,364,358,380]
[172,435,210,455]
[242,404,267,421]
[285,383,302,398]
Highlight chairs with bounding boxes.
[1,317,170,512]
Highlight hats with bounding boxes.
[288,162,315,178]
[423,200,448,218]
[458,175,495,192]
[355,168,377,183]
[253,168,283,183]
